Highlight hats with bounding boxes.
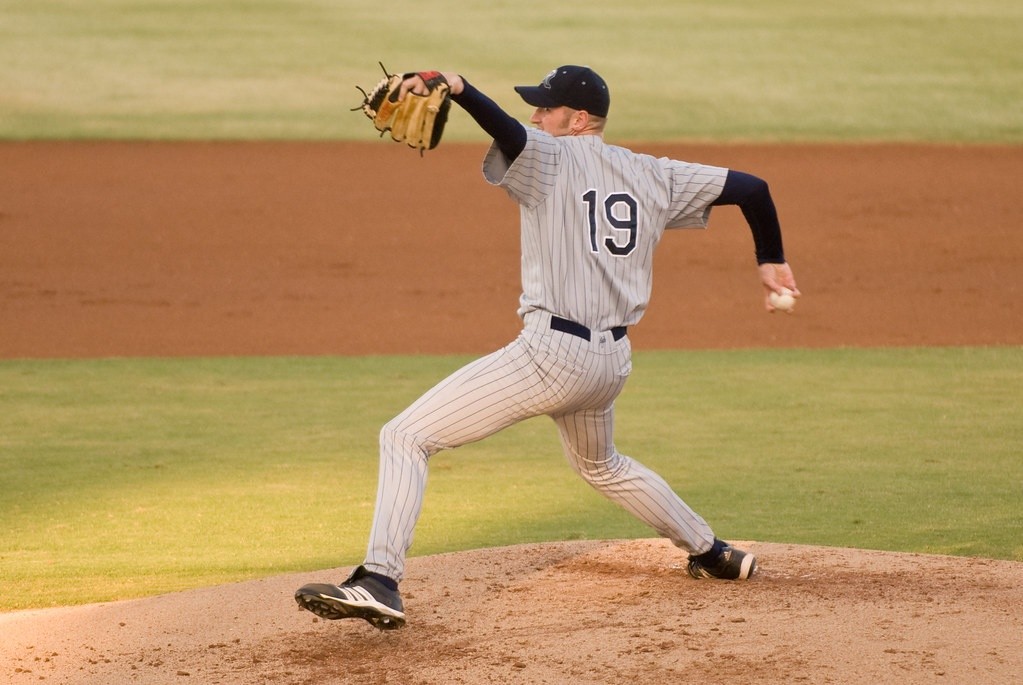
[514,65,610,118]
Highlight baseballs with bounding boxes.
[769,286,796,310]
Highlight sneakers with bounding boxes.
[687,540,758,579]
[294,565,406,633]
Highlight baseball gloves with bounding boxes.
[362,71,451,151]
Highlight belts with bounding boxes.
[550,316,626,342]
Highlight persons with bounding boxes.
[294,65,801,628]
